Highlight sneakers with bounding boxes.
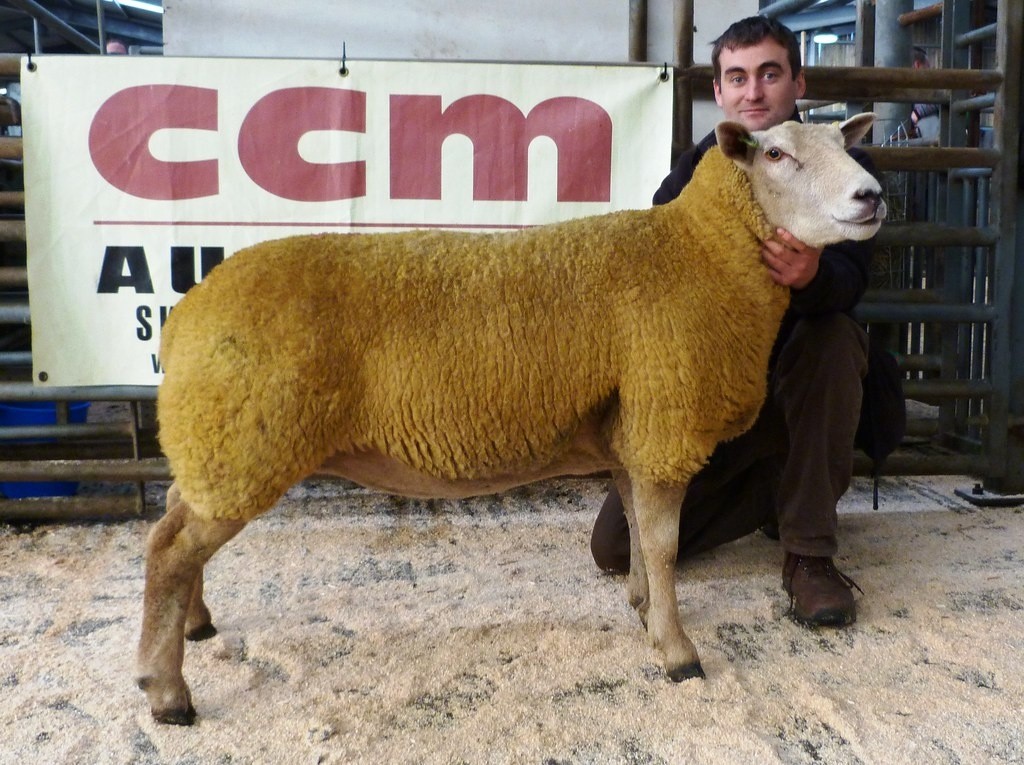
[762,519,782,541]
[779,542,863,624]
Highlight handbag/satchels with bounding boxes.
[855,348,906,509]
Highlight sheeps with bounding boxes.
[136,111,888,727]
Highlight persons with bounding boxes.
[911,47,939,122]
[590,17,877,625]
